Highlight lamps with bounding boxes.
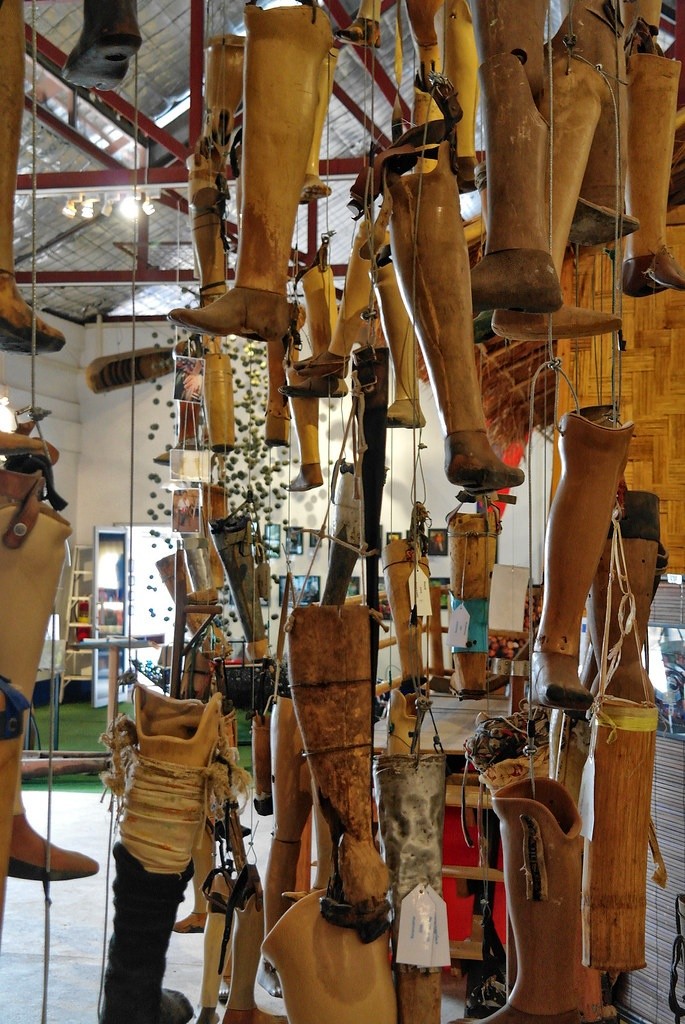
[59,191,155,218]
[152,95,191,128]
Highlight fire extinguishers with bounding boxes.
[74,595,91,641]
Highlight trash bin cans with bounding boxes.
[445,773,507,960]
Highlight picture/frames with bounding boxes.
[229,519,450,621]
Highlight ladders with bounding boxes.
[58,545,94,703]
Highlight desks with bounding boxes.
[295,688,528,962]
